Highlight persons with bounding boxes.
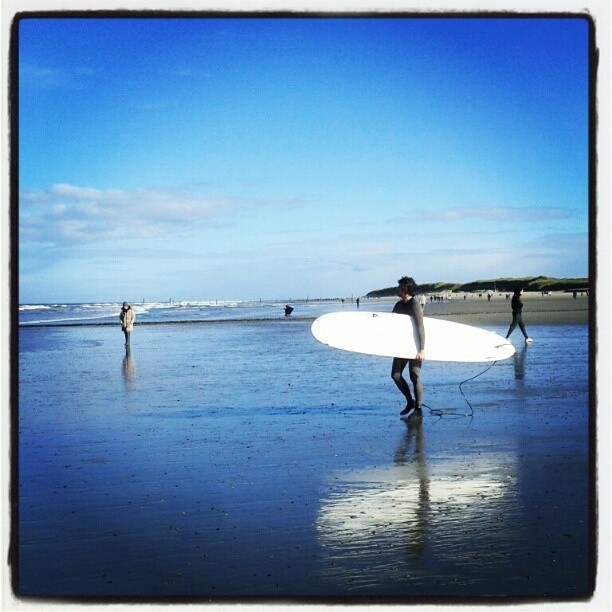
[419,294,428,309]
[487,294,491,302]
[391,276,426,422]
[463,296,468,301]
[428,292,446,303]
[449,296,452,302]
[356,296,360,308]
[283,305,294,317]
[506,285,534,343]
[119,301,135,347]
[505,294,509,301]
[509,293,512,300]
[541,288,589,299]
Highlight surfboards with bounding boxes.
[311,311,515,362]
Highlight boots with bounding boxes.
[409,407,423,419]
[400,398,416,415]
